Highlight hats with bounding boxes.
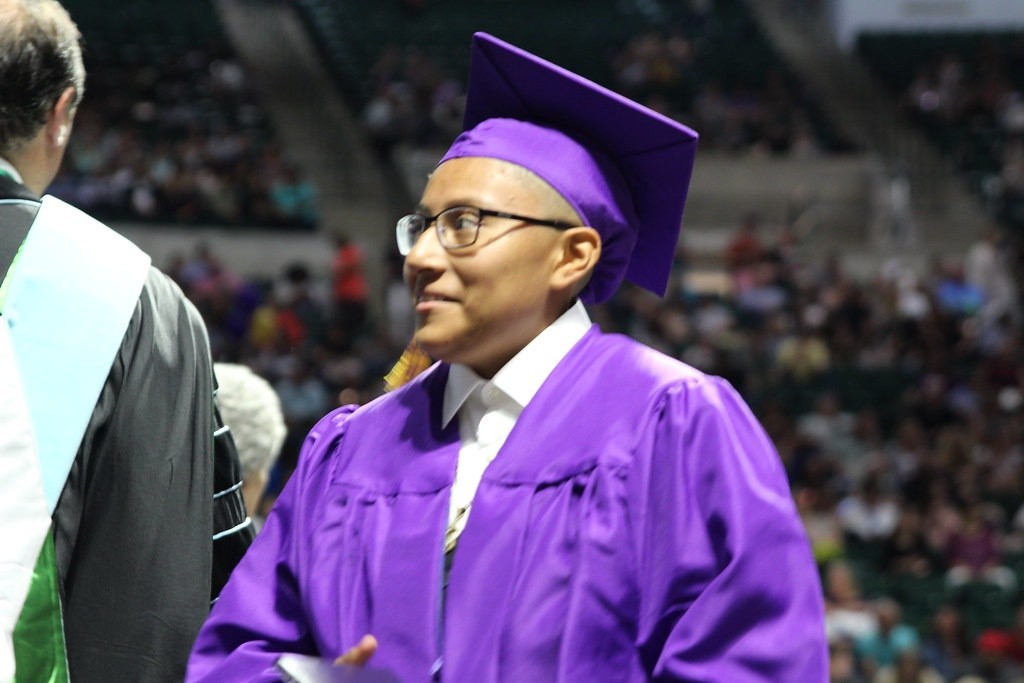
[382,33,698,393]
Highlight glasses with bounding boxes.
[396,205,580,256]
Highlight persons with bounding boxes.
[0,0,1024,683]
